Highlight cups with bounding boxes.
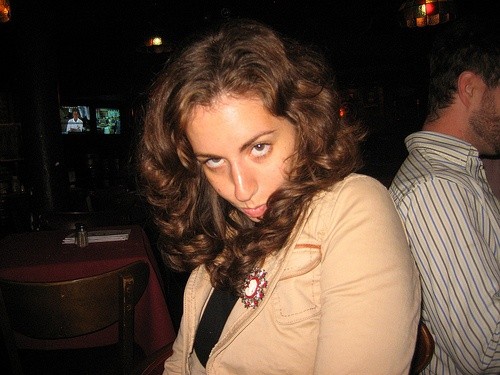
[76,223,90,249]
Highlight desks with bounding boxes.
[0,231,176,357]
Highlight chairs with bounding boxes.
[0,211,436,375]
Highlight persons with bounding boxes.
[66,108,84,133]
[390,28,500,375]
[135,18,423,375]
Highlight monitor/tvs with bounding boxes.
[59,105,91,135]
[96,108,121,135]
